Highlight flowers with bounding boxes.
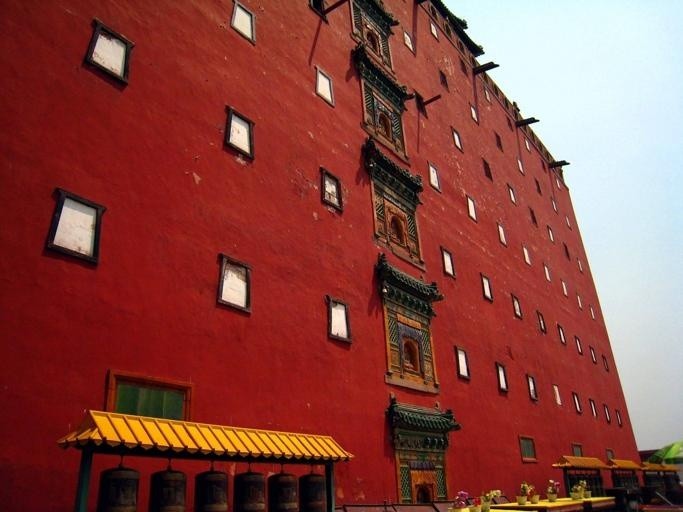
[481,490,502,502]
[571,480,587,492]
[471,498,481,505]
[451,491,468,508]
[520,480,535,496]
[545,479,560,494]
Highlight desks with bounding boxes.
[490,497,615,512]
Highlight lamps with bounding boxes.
[381,280,389,294]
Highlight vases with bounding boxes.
[448,507,469,512]
[481,503,490,512]
[516,496,527,505]
[584,491,592,497]
[547,494,557,502]
[569,492,580,499]
[531,495,540,504]
[468,505,482,512]
